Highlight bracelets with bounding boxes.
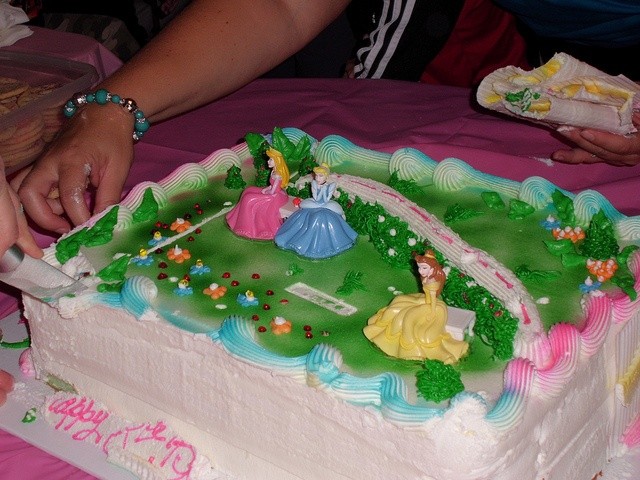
[63,88,150,144]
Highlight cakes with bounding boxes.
[18,126,636,478]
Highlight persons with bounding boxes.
[8,0,639,235]
[273,160,359,260]
[0,152,45,262]
[222,143,292,240]
[362,248,467,365]
